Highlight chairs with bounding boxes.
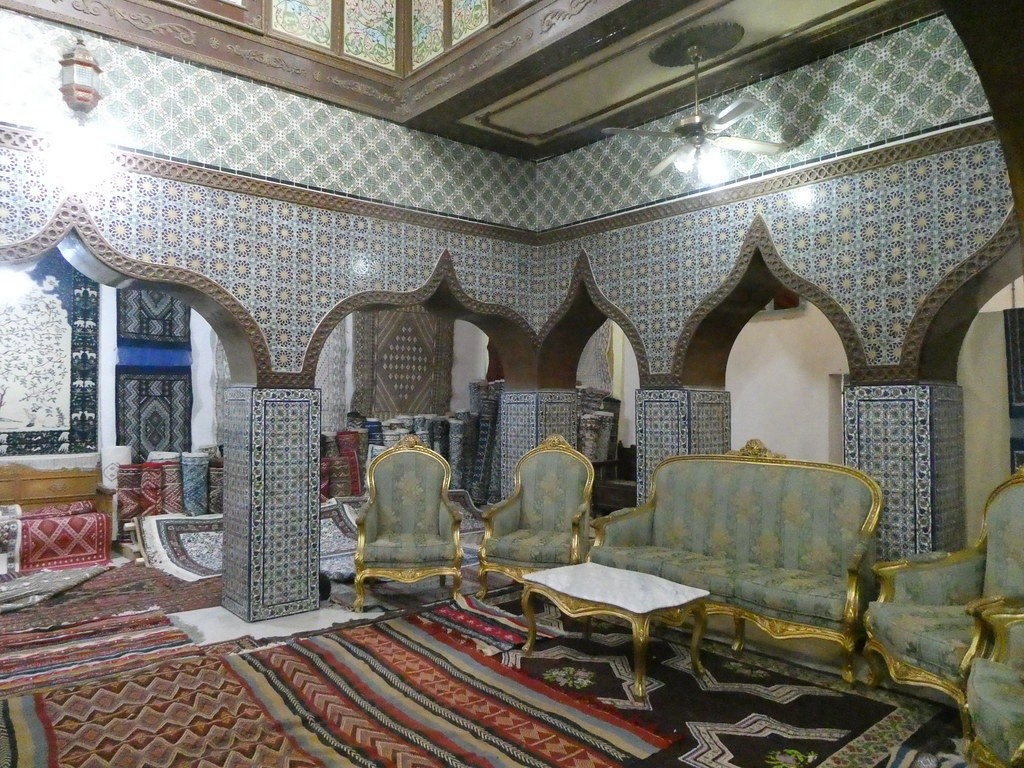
[861,461,1024,755]
[477,433,595,600]
[963,608,1024,768]
[353,433,468,612]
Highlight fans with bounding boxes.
[600,21,785,177]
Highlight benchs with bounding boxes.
[585,439,881,686]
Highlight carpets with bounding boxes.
[0,505,973,768]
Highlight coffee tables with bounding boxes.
[520,559,713,700]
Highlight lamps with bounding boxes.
[59,38,102,113]
